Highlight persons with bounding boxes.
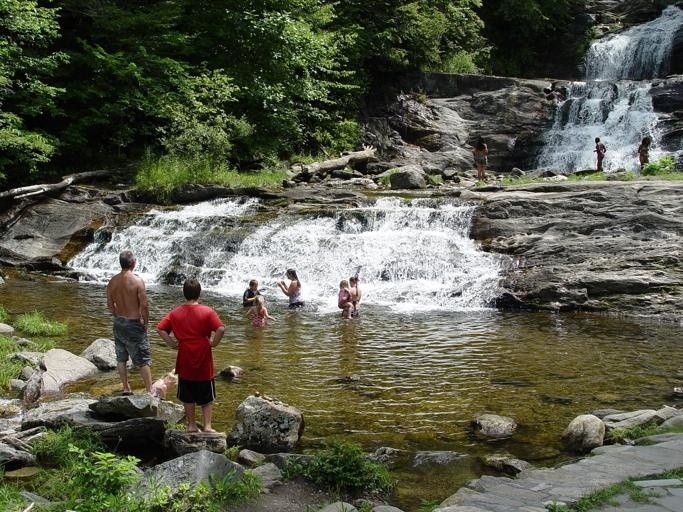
[156,278,226,433]
[245,295,277,327]
[277,268,305,309]
[638,137,650,170]
[337,279,354,320]
[475,137,490,181]
[341,276,362,317]
[106,250,158,398]
[243,279,265,307]
[593,137,606,171]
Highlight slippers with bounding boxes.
[122,391,134,396]
